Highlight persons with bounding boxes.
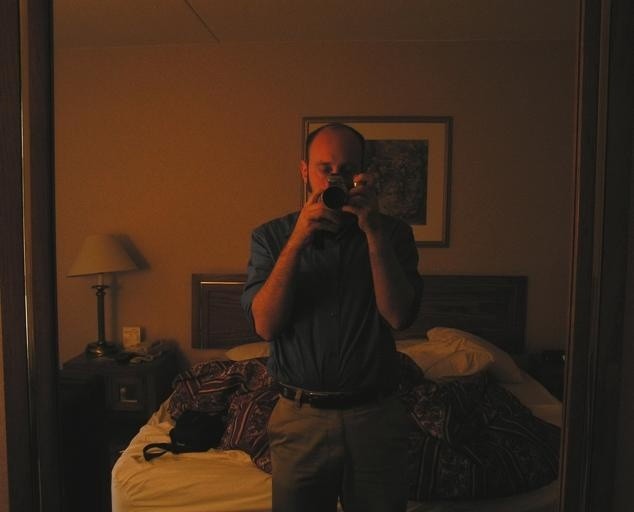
[240,124,424,512]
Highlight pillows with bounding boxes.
[394,326,524,386]
[224,341,269,362]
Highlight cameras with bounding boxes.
[316,175,357,223]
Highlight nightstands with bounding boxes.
[56,338,178,509]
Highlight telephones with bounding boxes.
[135,340,170,357]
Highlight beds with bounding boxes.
[109,270,564,511]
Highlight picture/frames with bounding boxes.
[299,114,453,250]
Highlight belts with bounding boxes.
[278,383,381,408]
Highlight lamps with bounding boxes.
[66,234,141,358]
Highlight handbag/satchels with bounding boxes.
[171,412,221,450]
